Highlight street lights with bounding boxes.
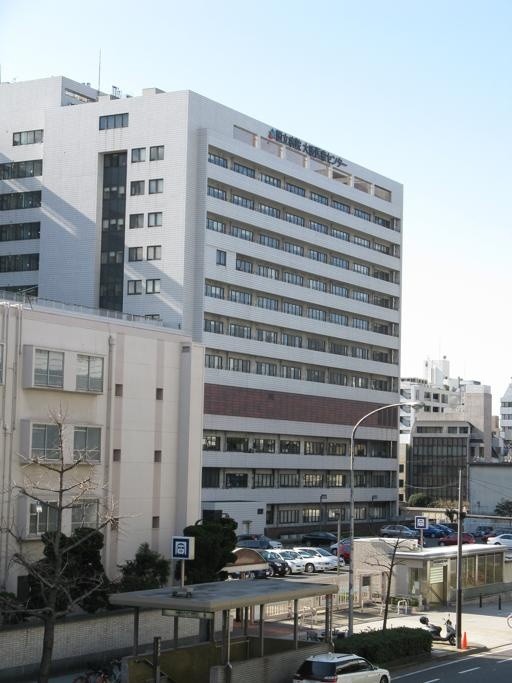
[348,401,425,637]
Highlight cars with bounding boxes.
[235,534,361,576]
[379,524,418,538]
[409,524,511,548]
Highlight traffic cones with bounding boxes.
[462,632,468,649]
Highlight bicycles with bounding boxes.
[74,659,121,683]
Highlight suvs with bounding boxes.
[293,651,391,683]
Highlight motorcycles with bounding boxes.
[417,615,458,646]
